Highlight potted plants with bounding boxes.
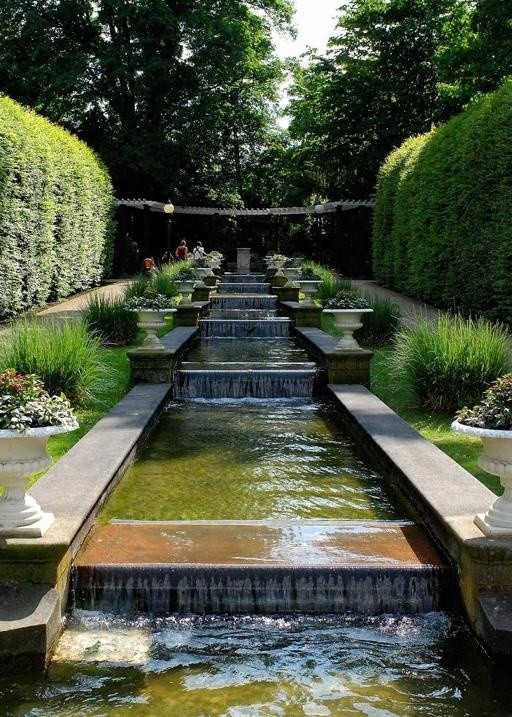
[0,365,84,540]
[320,288,375,352]
[124,257,212,351]
[260,247,323,304]
[448,371,512,538]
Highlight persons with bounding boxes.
[175,240,188,260]
[193,241,208,259]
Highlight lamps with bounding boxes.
[163,199,176,217]
[313,204,326,223]
